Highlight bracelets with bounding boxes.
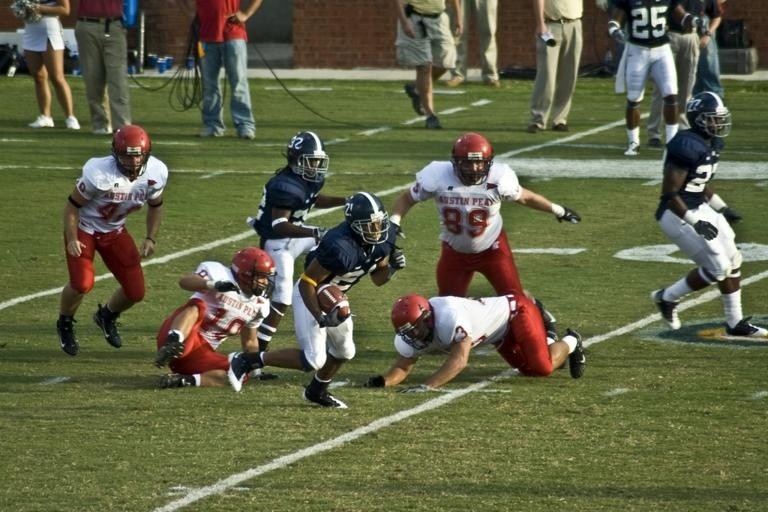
[551,203,566,217]
[367,376,386,388]
[207,280,217,291]
[249,369,262,376]
[147,236,156,244]
[388,214,402,226]
[318,314,325,327]
[387,264,396,280]
[707,195,727,213]
[684,210,698,226]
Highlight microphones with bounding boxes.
[540,31,558,47]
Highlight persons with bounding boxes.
[57,125,169,356]
[194,0,266,139]
[443,0,499,86]
[394,0,463,131]
[227,192,405,409]
[245,131,349,379]
[75,1,132,135]
[389,131,582,348]
[608,1,724,156]
[651,91,768,338]
[347,292,587,393]
[154,246,279,388]
[16,0,80,130]
[530,0,584,133]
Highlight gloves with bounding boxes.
[361,375,386,388]
[391,215,406,240]
[551,202,581,224]
[605,20,620,35]
[389,249,406,269]
[682,210,717,240]
[206,279,241,295]
[680,13,703,29]
[253,367,278,380]
[397,385,431,394]
[317,306,351,327]
[706,196,742,224]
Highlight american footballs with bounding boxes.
[315,282,350,319]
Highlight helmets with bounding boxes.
[233,247,277,298]
[289,132,329,182]
[344,193,389,244]
[391,296,434,349]
[112,125,151,177]
[451,132,493,184]
[686,92,733,137]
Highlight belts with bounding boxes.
[79,18,122,37]
[412,11,441,18]
[668,26,692,34]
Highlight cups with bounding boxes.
[185,57,194,69]
[146,53,175,73]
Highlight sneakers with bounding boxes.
[528,124,540,133]
[533,298,585,377]
[486,78,501,88]
[153,331,185,367]
[651,288,681,330]
[406,83,427,115]
[28,115,54,128]
[551,122,568,131]
[93,303,122,347]
[648,138,661,147]
[425,116,443,129]
[159,373,196,387]
[227,351,250,392]
[66,116,80,130]
[726,317,768,337]
[303,373,346,408]
[625,142,641,156]
[444,79,465,87]
[57,314,79,356]
